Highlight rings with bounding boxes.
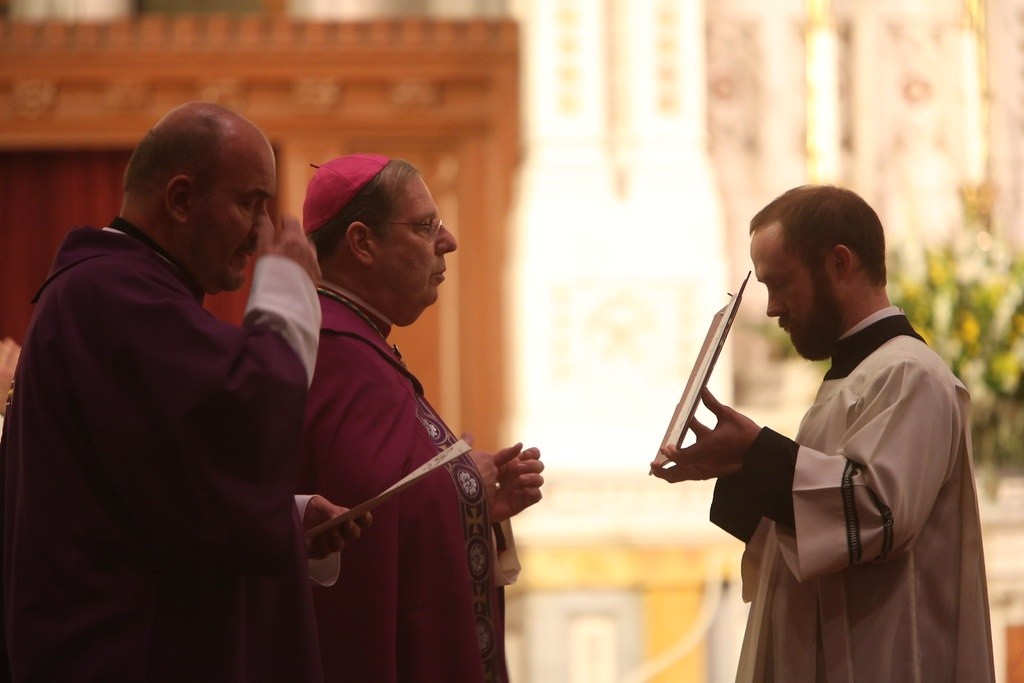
[494,482,502,492]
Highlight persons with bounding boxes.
[651,185,996,683]
[0,101,373,683]
[301,155,545,683]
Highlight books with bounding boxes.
[648,270,751,476]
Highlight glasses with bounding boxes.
[364,216,444,244]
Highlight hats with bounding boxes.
[302,154,394,238]
